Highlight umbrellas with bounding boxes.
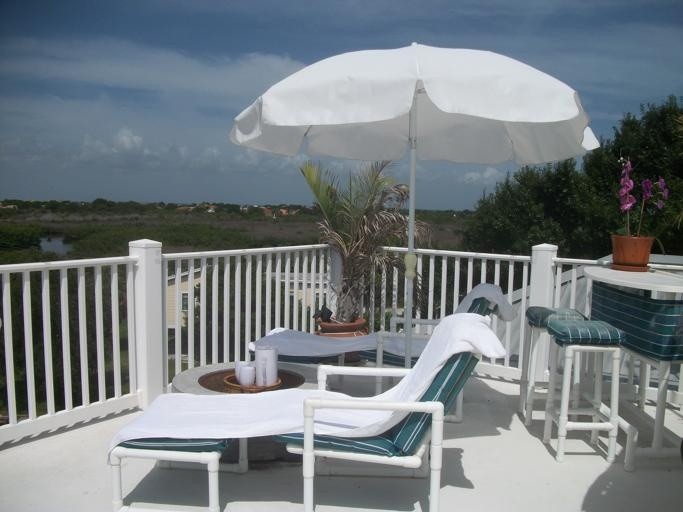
[226,42,601,371]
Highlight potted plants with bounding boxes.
[295,155,431,362]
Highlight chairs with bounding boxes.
[248,283,518,422]
[107,312,507,512]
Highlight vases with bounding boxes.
[608,234,655,271]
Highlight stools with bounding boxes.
[542,319,626,464]
[518,306,589,427]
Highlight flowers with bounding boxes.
[617,155,669,237]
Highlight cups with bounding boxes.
[235,361,255,386]
[255,347,278,386]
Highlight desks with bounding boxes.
[169,363,329,462]
[584,265,683,472]
